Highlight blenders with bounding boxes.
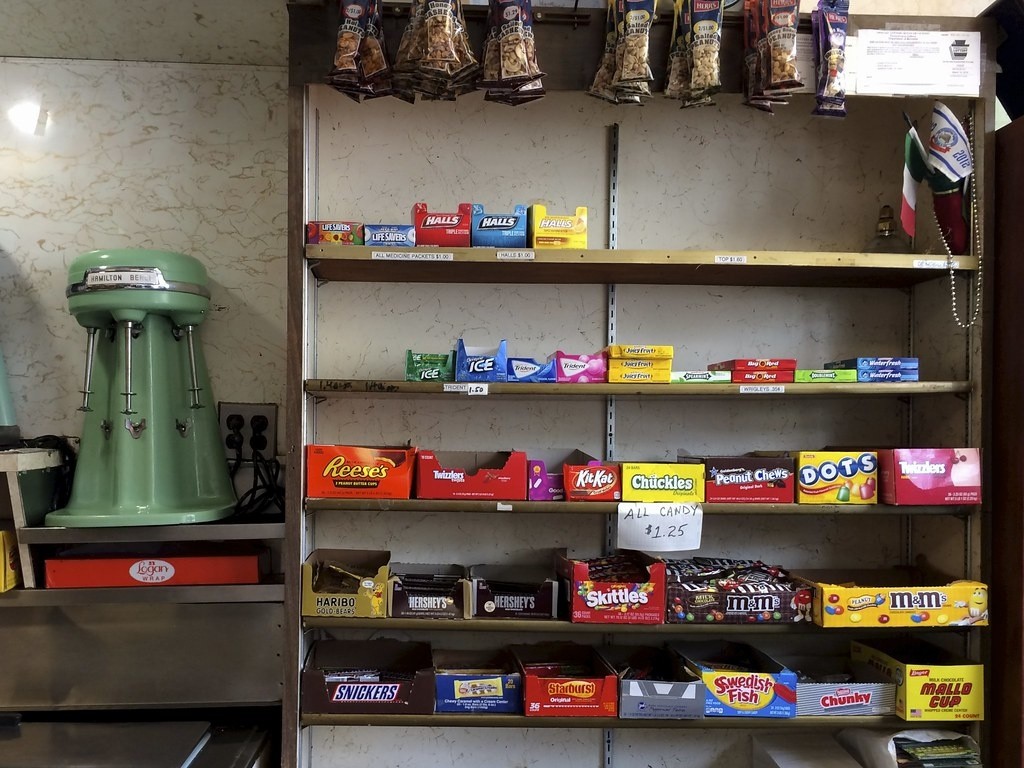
[44,246,240,525]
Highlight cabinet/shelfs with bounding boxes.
[282,0,1002,768]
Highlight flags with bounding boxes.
[900,127,933,237]
[929,99,973,181]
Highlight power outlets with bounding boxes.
[219,402,277,461]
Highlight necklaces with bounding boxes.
[931,114,982,328]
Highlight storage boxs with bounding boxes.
[388,562,472,620]
[302,549,391,618]
[770,655,896,717]
[406,339,920,383]
[666,585,814,625]
[851,639,985,721]
[43,549,271,589]
[302,640,434,715]
[604,461,705,503]
[825,446,982,506]
[676,456,794,504]
[306,198,589,250]
[595,645,705,720]
[526,448,602,501]
[416,449,527,501]
[785,567,989,628]
[306,445,416,499]
[509,642,618,718]
[552,547,664,625]
[470,564,558,619]
[664,639,797,718]
[754,450,878,505]
[433,649,522,714]
